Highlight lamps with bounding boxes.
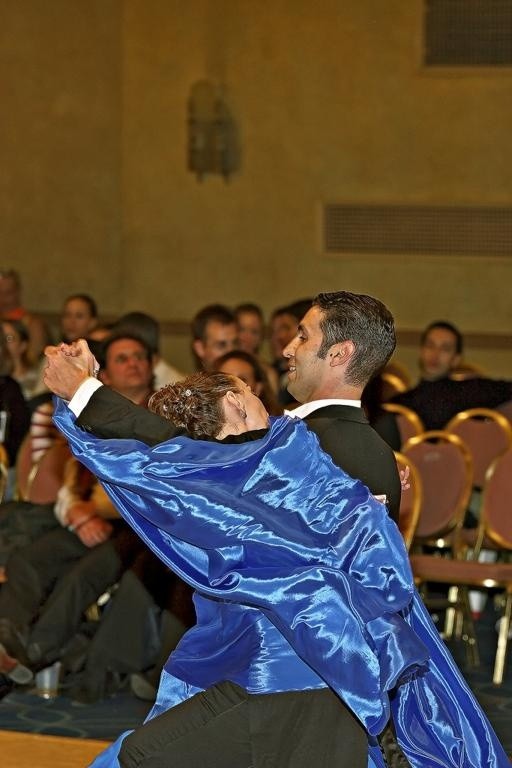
[187,79,237,183]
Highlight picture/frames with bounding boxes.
[412,0,512,76]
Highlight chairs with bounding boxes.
[409,450,511,684]
[381,402,424,447]
[392,451,421,552]
[400,431,480,667]
[430,410,511,547]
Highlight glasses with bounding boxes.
[115,350,147,364]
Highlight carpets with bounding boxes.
[0,617,511,756]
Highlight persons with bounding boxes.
[190,303,241,378]
[1,317,36,379]
[269,308,300,405]
[59,294,102,345]
[1,333,162,700]
[41,338,512,768]
[213,349,264,402]
[231,302,264,356]
[42,291,403,768]
[1,269,35,335]
[378,318,512,448]
[111,312,189,397]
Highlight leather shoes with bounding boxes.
[0,644,33,685]
[131,663,163,701]
